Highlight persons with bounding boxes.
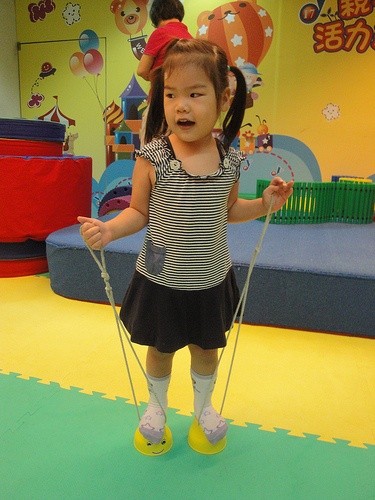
[78,38,294,446]
[137,0,194,150]
